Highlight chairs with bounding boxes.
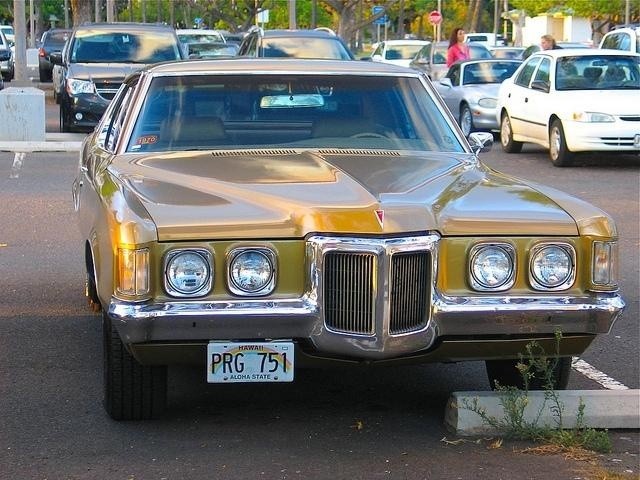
[308,114,378,141]
[144,115,237,150]
[554,61,629,90]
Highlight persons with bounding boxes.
[446,28,472,69]
[541,32,566,50]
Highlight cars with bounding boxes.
[73,56,625,422]
[370,25,640,167]
[0,24,16,87]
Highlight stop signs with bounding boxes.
[429,11,443,24]
